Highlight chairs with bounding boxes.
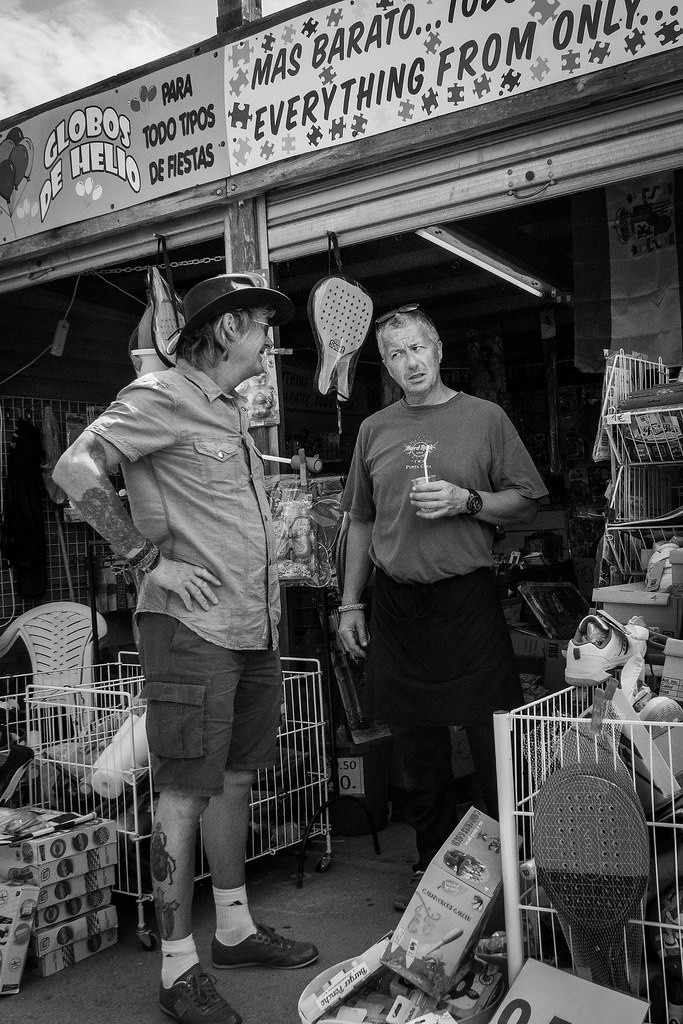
[0,601,110,734]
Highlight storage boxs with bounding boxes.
[380,806,525,1001]
[0,809,120,995]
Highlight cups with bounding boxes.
[411,475,438,514]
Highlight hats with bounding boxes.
[166,273,294,357]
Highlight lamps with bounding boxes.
[415,226,566,305]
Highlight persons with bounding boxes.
[335,303,555,913]
[50,275,319,1024]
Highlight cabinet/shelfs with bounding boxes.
[491,346,683,1024]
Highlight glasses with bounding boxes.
[243,318,270,337]
[375,303,434,343]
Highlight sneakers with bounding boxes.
[212,920,320,969]
[158,959,244,1023]
[392,868,424,910]
[611,687,683,739]
[645,543,681,593]
[565,614,646,685]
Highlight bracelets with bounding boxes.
[338,603,367,612]
[128,538,162,575]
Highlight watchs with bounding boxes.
[464,488,483,516]
